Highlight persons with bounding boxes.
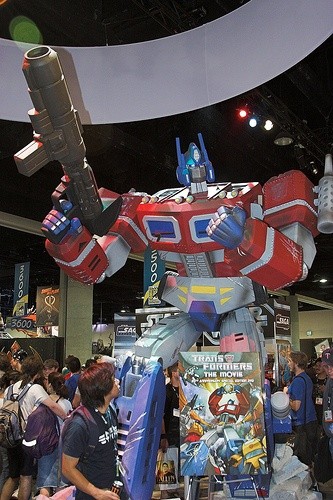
[283,349,333,491]
[157,463,176,482]
[0,350,102,500]
[40,132,316,500]
[61,362,121,500]
[160,364,180,449]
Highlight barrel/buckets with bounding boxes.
[270,392,289,419]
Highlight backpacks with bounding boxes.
[0,382,33,449]
[22,397,60,457]
[58,406,97,482]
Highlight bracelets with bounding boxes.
[161,433,167,440]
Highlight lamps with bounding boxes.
[239,105,293,146]
[310,161,318,174]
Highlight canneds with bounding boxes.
[110,480,123,496]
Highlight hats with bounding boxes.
[321,349,333,367]
